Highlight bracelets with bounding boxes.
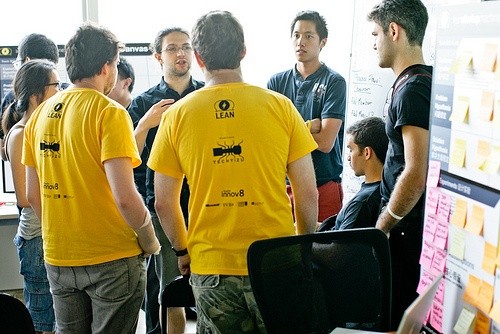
[307,119,312,132]
[385,205,403,221]
[132,206,152,231]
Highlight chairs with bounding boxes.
[247,227,393,334]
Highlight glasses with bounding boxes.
[12,59,26,69]
[159,44,195,54]
[45,81,61,90]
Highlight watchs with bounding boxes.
[172,247,188,257]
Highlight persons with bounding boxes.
[366,0,434,332]
[266,12,346,224]
[0,23,205,334]
[147,11,318,334]
[312,117,389,272]
